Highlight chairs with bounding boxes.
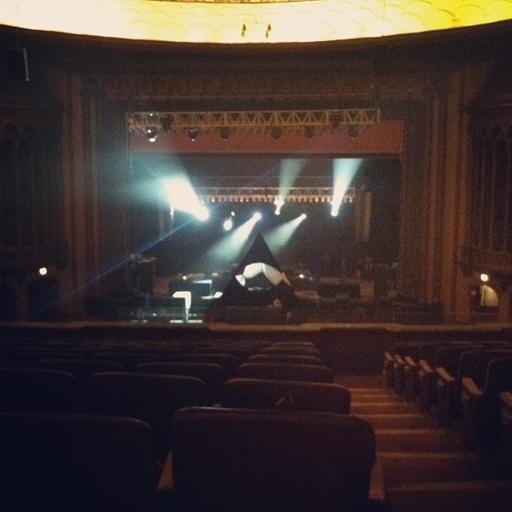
[381,330,512,488]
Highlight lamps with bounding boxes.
[140,110,359,143]
